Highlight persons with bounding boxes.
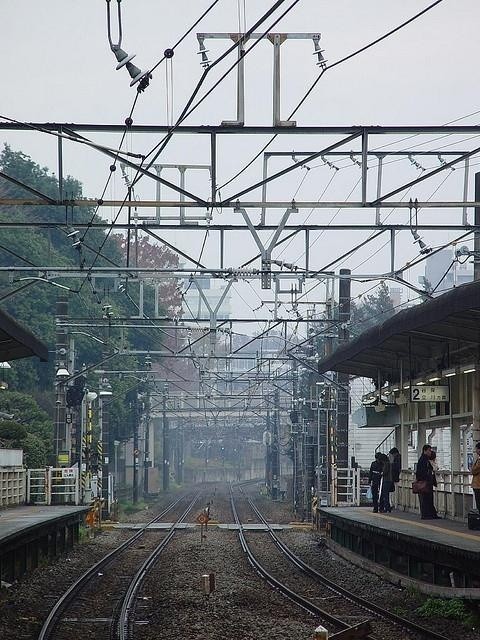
[415,445,442,520]
[387,447,402,482]
[428,451,440,514]
[368,452,386,513]
[470,442,480,515]
[377,454,393,513]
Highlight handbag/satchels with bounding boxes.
[412,481,430,494]
[468,509,479,530]
[383,480,394,492]
